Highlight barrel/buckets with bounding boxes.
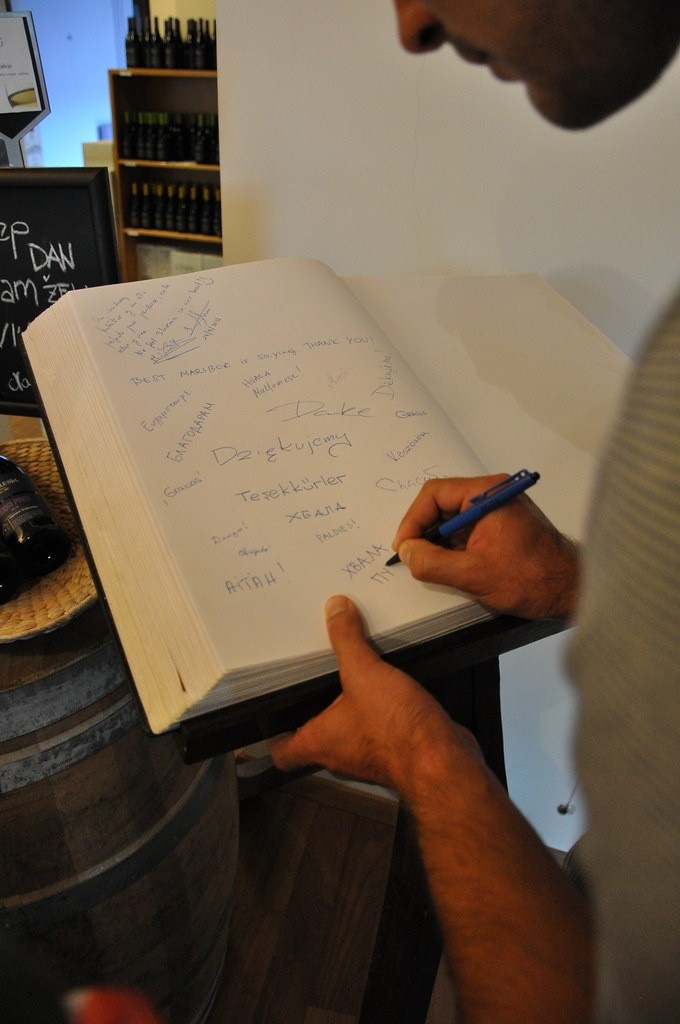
[1,602,243,1024]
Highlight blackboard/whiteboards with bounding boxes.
[0,166,121,415]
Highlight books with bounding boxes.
[22,258,645,769]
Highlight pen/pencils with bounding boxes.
[384,469,541,567]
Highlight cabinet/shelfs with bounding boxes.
[108,69,223,285]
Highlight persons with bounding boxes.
[260,0,680,1023]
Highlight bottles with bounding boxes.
[128,180,222,236]
[118,110,220,166]
[0,454,71,606]
[125,15,218,69]
